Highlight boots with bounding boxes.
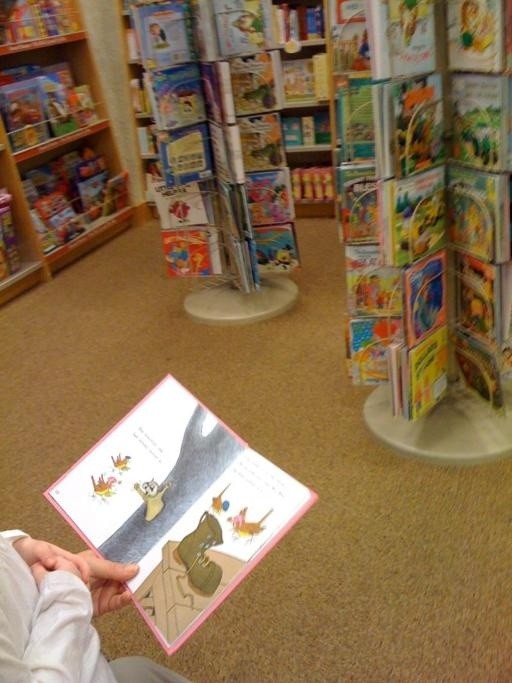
[173,512,225,596]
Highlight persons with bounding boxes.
[0,529,193,683]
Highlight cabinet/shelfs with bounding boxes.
[116,1,340,219]
[0,4,144,302]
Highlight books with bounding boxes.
[1,0,512,422]
[42,373,319,657]
